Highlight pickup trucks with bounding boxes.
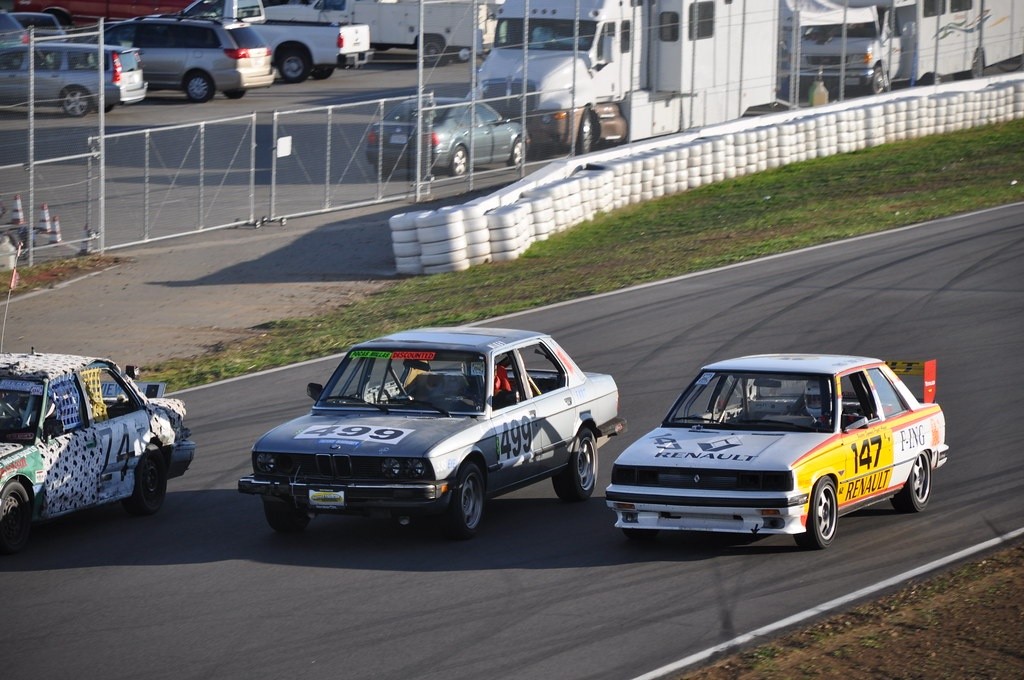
[177,0,370,84]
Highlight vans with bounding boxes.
[0,42,150,118]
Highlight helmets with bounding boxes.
[803,379,833,418]
[16,392,37,423]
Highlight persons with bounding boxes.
[798,380,832,421]
[16,391,40,428]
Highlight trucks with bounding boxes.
[465,1,781,156]
[264,0,483,69]
[777,0,1023,96]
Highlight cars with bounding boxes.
[604,351,948,550]
[0,8,69,43]
[236,324,627,541]
[0,347,197,557]
[72,13,277,104]
[365,96,533,177]
